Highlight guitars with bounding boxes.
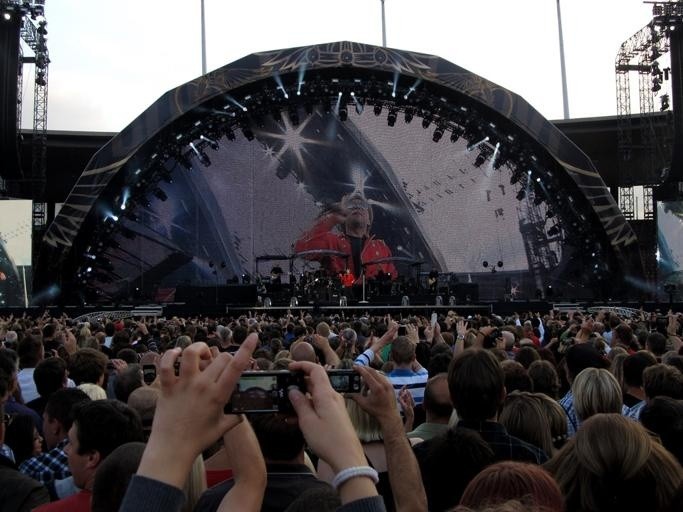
[429,275,446,285]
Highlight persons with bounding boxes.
[292,189,397,278]
[337,268,355,298]
[270,264,282,287]
[370,265,384,289]
[1,304,682,508]
[428,266,438,293]
[314,266,326,287]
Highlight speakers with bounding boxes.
[217,284,258,307]
[175,286,216,306]
[450,283,480,306]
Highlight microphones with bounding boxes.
[325,200,353,216]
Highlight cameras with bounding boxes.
[222,368,299,415]
[327,369,361,394]
[143,364,156,382]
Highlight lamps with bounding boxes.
[492,146,611,297]
[177,92,493,172]
[81,159,177,299]
